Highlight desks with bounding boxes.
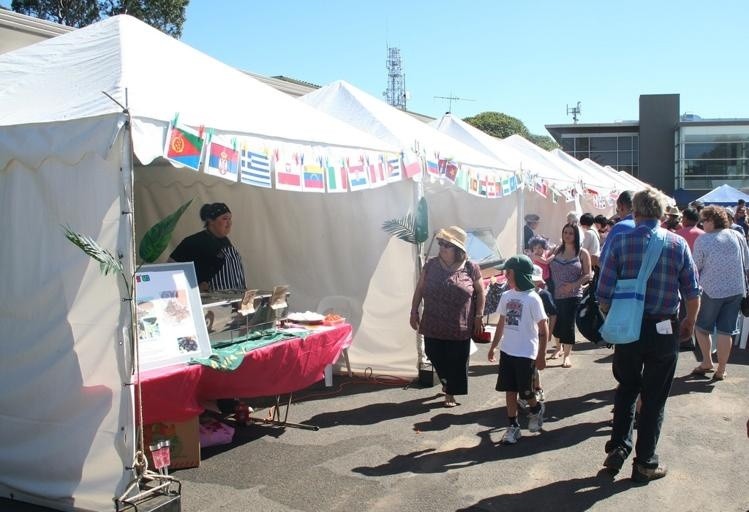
[135,318,352,430]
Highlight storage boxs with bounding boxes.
[135,411,200,470]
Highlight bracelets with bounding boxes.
[475,314,484,320]
[408,309,419,316]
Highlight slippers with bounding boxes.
[712,368,727,380]
[444,399,457,406]
[692,366,713,373]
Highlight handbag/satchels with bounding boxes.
[199,417,235,448]
[576,281,614,348]
[741,293,749,317]
[471,332,490,343]
[598,279,647,344]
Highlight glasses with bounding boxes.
[699,218,709,227]
[438,242,454,249]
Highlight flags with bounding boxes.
[239,150,270,185]
[165,126,203,168]
[369,165,384,185]
[329,160,347,189]
[344,164,366,186]
[208,142,237,174]
[304,163,323,187]
[277,158,302,186]
[388,155,618,209]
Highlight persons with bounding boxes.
[407,225,488,407]
[487,187,749,442]
[595,189,702,484]
[166,200,245,291]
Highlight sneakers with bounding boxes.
[518,399,529,407]
[632,463,667,484]
[603,448,628,470]
[527,402,545,433]
[501,424,522,445]
[535,389,544,401]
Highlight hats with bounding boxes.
[494,254,536,291]
[531,263,543,281]
[726,207,736,217]
[436,225,467,253]
[664,206,685,217]
[525,215,540,221]
